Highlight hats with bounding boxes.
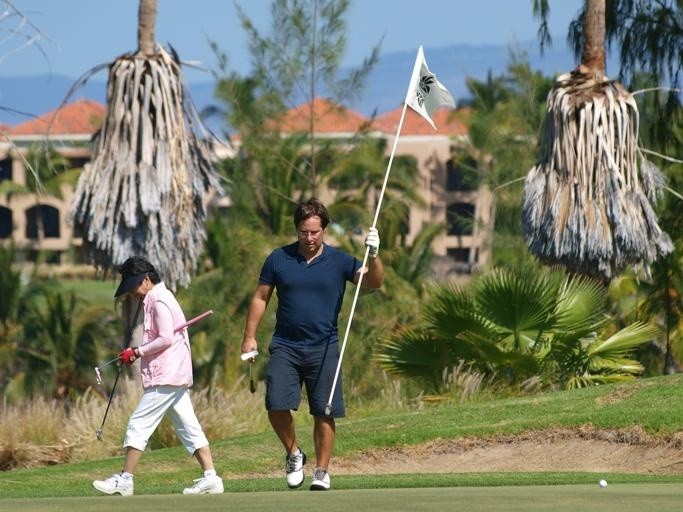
[112,267,156,297]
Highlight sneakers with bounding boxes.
[92,472,135,498]
[307,468,331,491]
[182,474,225,495]
[283,446,307,489]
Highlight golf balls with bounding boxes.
[598,479,608,489]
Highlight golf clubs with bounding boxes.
[94,310,213,385]
[96,294,144,444]
[240,350,259,394]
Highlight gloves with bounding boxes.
[361,226,381,260]
[116,347,138,368]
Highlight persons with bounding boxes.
[88,255,226,498]
[239,198,385,493]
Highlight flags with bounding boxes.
[402,43,457,133]
